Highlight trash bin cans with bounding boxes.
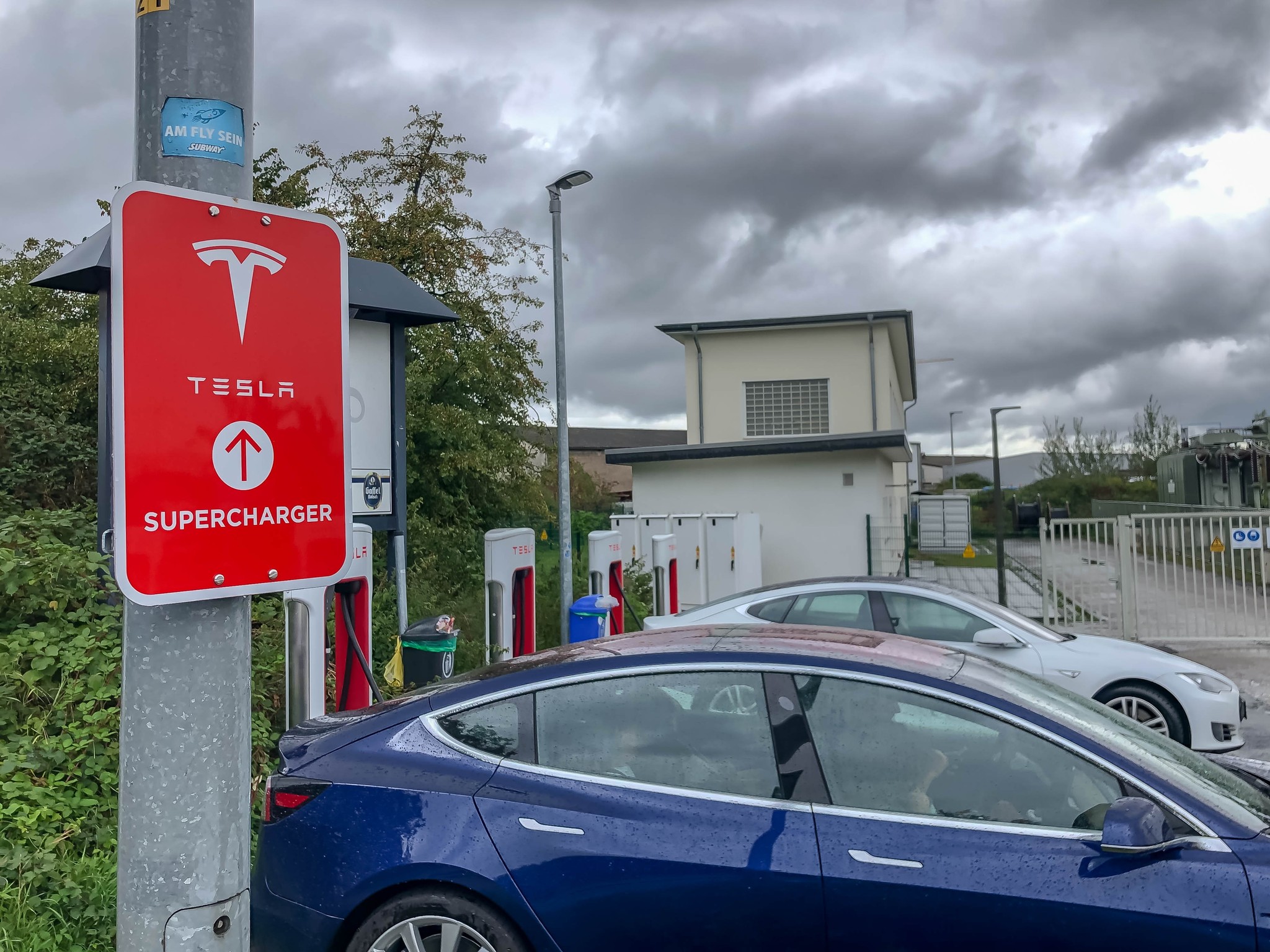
[568,593,613,643]
[401,615,461,694]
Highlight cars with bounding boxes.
[641,574,1249,754]
[249,619,1270,952]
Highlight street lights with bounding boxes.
[949,410,964,494]
[989,405,1021,607]
[544,169,596,639]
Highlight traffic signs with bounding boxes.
[111,179,352,607]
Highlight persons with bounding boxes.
[832,695,1023,825]
